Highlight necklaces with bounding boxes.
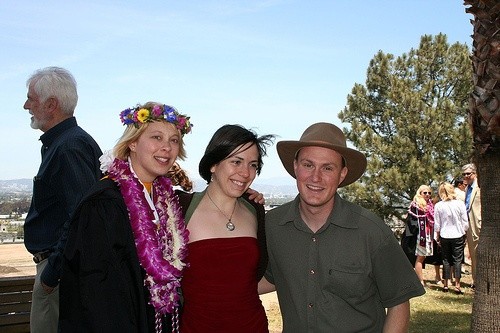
[102,158,191,312]
[206,187,238,231]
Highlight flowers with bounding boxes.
[120,105,194,133]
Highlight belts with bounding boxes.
[33,251,51,264]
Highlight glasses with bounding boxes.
[423,192,431,195]
[462,172,471,176]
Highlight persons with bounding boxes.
[258,123,426,333]
[175,125,270,333]
[23,67,107,333]
[400,163,482,295]
[59,102,194,333]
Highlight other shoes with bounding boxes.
[443,286,448,292]
[452,288,464,295]
[435,275,441,285]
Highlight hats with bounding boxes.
[276,122,367,188]
